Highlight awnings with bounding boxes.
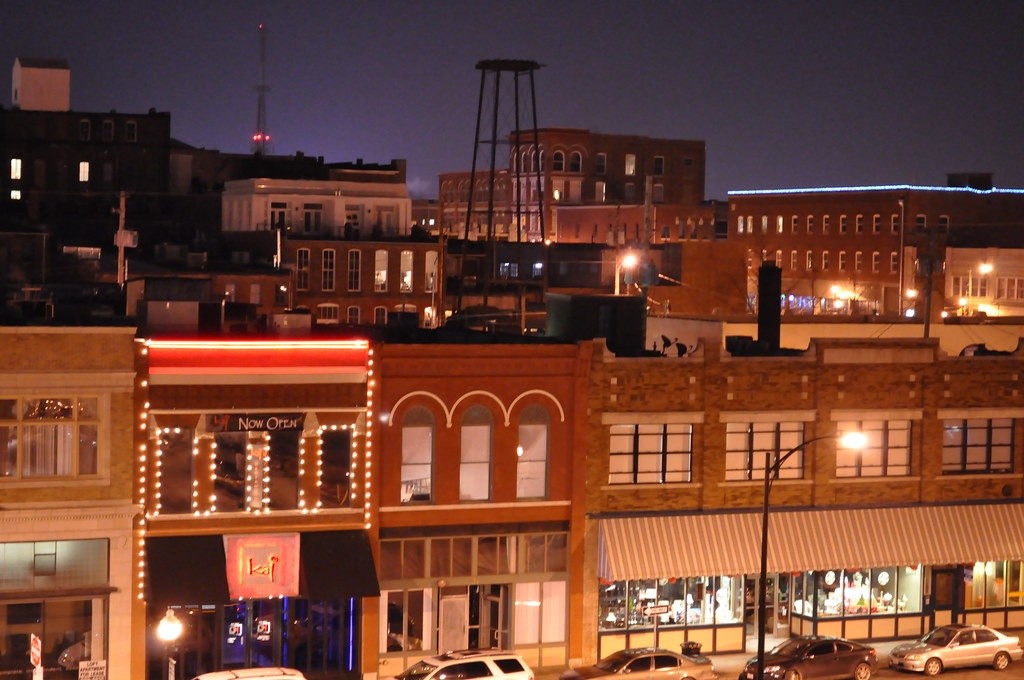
[143,528,381,606]
[598,502,1024,586]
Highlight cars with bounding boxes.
[192,666,306,680]
[739,632,879,680]
[558,647,716,680]
[888,623,1024,676]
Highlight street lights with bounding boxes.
[615,254,636,295]
[758,430,869,680]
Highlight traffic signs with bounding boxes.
[642,606,669,617]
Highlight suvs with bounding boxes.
[380,649,535,680]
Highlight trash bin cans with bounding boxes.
[681,642,702,657]
